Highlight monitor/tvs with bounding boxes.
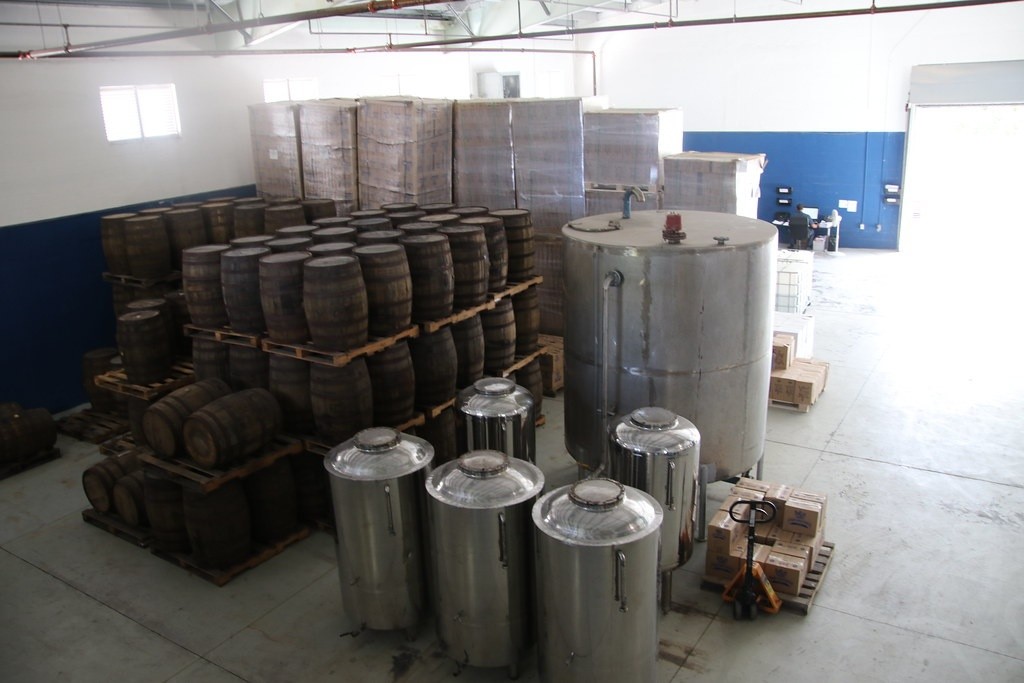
[802,208,819,220]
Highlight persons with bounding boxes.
[785,204,818,248]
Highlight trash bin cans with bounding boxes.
[828,236,837,251]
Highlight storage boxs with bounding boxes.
[769,334,829,405]
[249,96,766,333]
[537,333,564,392]
[706,477,827,596]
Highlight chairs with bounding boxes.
[788,217,809,252]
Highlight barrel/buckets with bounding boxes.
[0,400,57,474]
[79,198,544,573]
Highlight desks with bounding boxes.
[772,219,839,252]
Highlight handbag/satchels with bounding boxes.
[828,236,835,251]
[775,212,791,222]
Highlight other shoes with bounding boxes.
[807,247,812,250]
[787,245,794,249]
